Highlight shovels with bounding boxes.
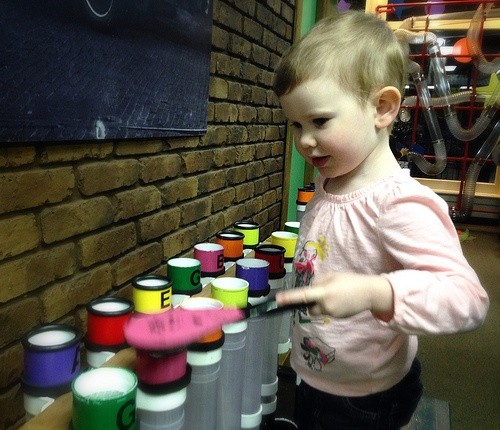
[125,292,314,352]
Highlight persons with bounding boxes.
[272,9,490,430]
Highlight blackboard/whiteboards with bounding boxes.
[0,0,212,143]
